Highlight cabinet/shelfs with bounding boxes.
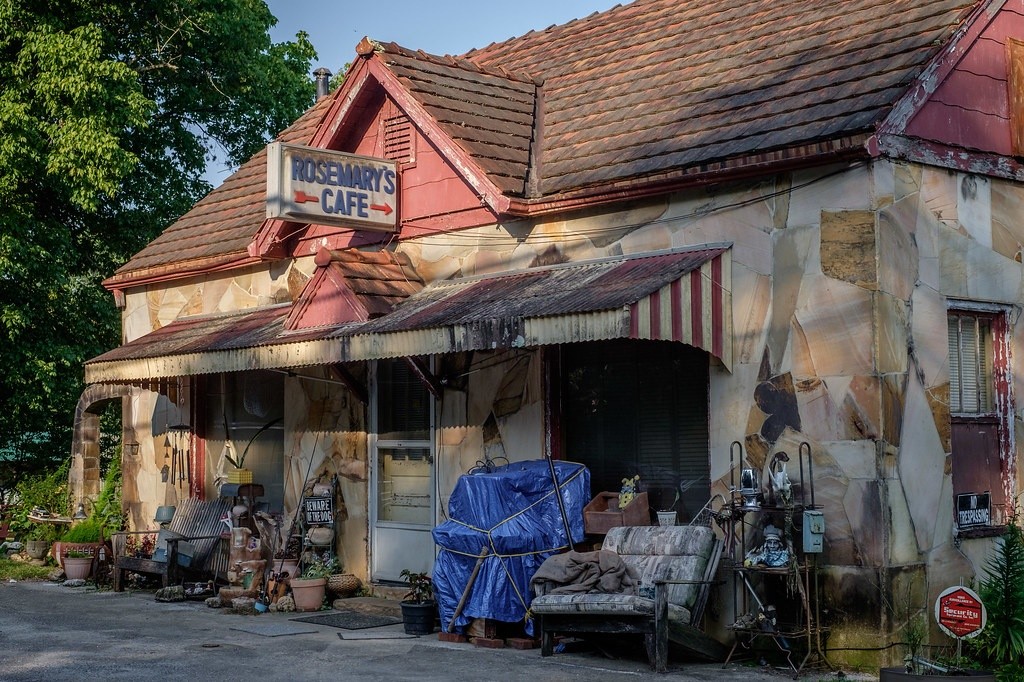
[722,438,836,678]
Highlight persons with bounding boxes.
[765,533,780,551]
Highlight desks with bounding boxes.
[26,514,73,538]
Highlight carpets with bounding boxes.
[287,611,407,630]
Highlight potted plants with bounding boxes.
[224,415,285,483]
[51,522,100,578]
[290,558,335,611]
[25,524,48,566]
[399,569,437,635]
[62,549,94,581]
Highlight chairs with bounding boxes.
[110,497,235,595]
[529,523,729,675]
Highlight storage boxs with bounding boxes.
[582,490,651,536]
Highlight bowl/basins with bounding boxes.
[155,505,176,524]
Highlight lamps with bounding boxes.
[74,497,98,520]
[125,430,139,456]
